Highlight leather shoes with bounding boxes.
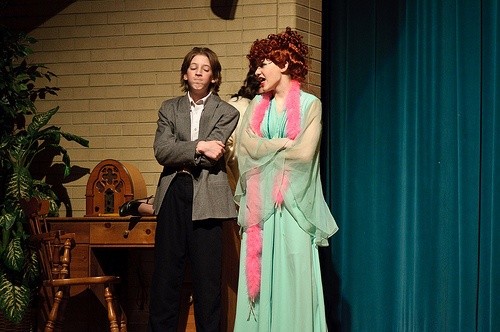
[119,195,153,216]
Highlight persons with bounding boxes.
[230,24,338,332]
[148,46,243,331]
[117,66,261,220]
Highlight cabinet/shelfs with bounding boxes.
[34,216,243,332]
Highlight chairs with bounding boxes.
[20,198,127,332]
